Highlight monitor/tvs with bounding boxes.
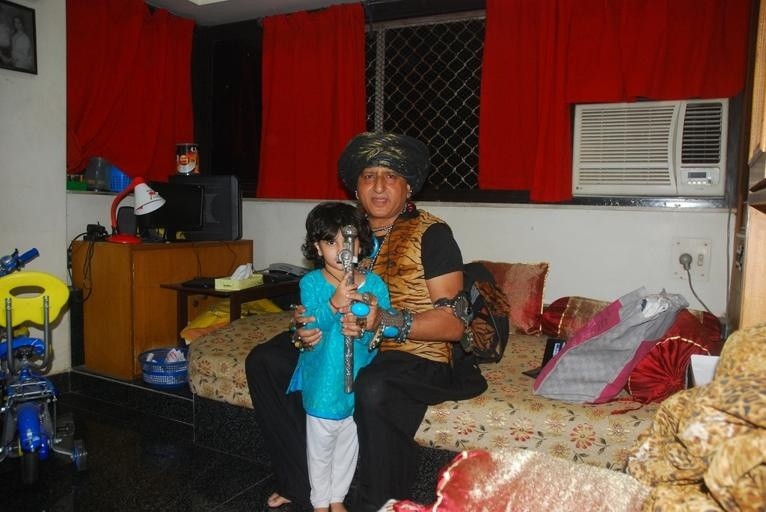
[143,180,204,243]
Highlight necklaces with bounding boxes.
[324,266,342,284]
[364,212,392,232]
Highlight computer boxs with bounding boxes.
[167,175,242,241]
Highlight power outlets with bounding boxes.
[671,238,711,283]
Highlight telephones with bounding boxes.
[268,263,309,277]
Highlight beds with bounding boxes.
[186,308,698,478]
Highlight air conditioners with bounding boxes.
[571,97,729,200]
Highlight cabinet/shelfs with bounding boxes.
[71,239,253,382]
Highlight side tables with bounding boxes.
[156,271,301,352]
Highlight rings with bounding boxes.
[289,304,299,313]
[357,293,371,339]
[289,325,306,353]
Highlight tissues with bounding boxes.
[215,263,265,292]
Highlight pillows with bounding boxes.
[540,296,609,340]
[476,261,548,340]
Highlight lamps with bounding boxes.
[105,175,167,244]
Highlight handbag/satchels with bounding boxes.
[531,286,688,406]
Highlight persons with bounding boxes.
[0,15,34,71]
[244,132,488,512]
[297,201,390,512]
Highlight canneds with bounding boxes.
[175,144,200,175]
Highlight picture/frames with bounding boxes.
[0,0,37,75]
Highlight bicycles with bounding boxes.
[0,248,91,483]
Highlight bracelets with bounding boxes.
[371,304,413,344]
[329,297,341,310]
